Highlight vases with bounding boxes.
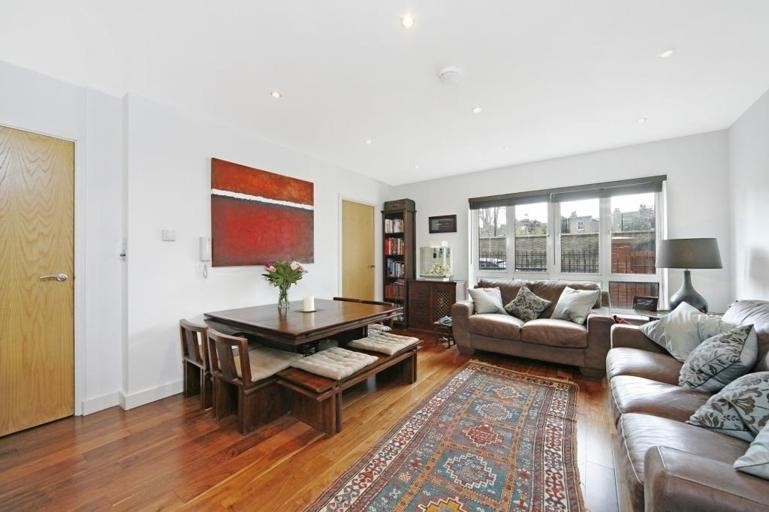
[277,285,290,309]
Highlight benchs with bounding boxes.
[275,339,425,435]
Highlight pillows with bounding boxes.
[504,283,551,321]
[550,286,599,325]
[638,301,769,481]
[468,287,509,315]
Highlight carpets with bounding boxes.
[302,358,586,512]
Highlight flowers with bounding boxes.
[262,258,308,299]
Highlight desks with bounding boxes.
[205,299,404,353]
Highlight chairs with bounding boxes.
[362,300,394,336]
[180,319,264,410]
[206,329,303,435]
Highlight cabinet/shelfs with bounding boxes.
[408,280,465,336]
[381,208,417,328]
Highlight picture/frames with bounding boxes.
[429,215,457,233]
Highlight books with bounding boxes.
[383,217,406,321]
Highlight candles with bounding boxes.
[304,296,315,312]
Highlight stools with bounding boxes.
[433,321,455,348]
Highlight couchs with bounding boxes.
[451,278,614,380]
[605,300,769,512]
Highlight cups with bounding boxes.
[303,295,315,312]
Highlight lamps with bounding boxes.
[655,238,723,314]
